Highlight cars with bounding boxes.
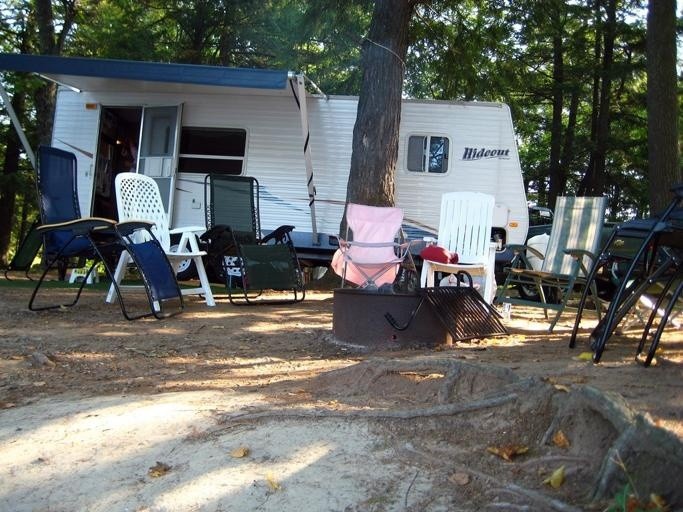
[513,205,682,302]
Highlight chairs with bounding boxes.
[5,145,217,321]
[199,173,305,306]
[330,202,423,289]
[492,195,608,331]
[568,184,683,367]
[420,191,497,315]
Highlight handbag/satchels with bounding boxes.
[419,243,459,266]
